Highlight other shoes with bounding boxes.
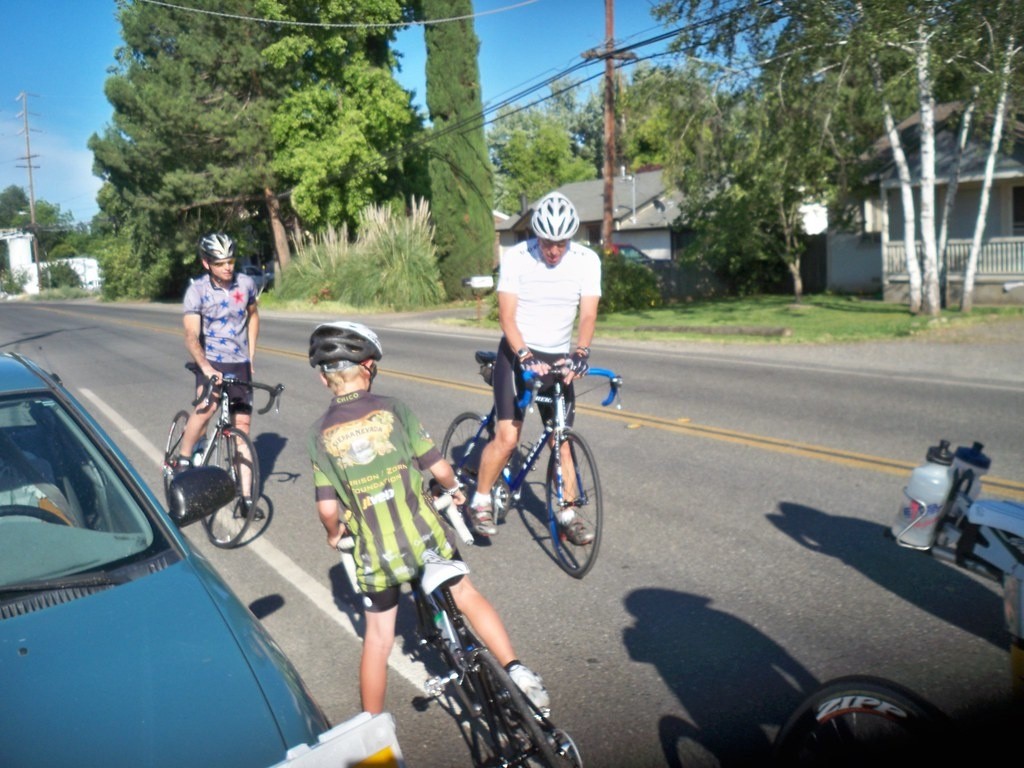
[240,496,266,520]
[509,665,551,722]
[168,451,197,473]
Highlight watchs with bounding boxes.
[516,347,532,359]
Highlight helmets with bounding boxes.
[309,320,383,367]
[199,233,235,259]
[530,191,580,243]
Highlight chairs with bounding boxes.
[16,463,80,528]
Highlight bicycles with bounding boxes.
[161,357,285,551]
[769,674,1003,766]
[331,479,587,767]
[439,350,623,580]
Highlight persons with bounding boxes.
[0,448,74,528]
[307,320,549,720]
[465,191,603,543]
[173,233,265,521]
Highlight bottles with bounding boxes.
[893,440,952,549]
[935,439,993,551]
[434,609,462,658]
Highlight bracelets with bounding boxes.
[577,346,590,356]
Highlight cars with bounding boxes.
[1,352,343,768]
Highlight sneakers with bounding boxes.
[463,503,499,538]
[557,515,594,546]
